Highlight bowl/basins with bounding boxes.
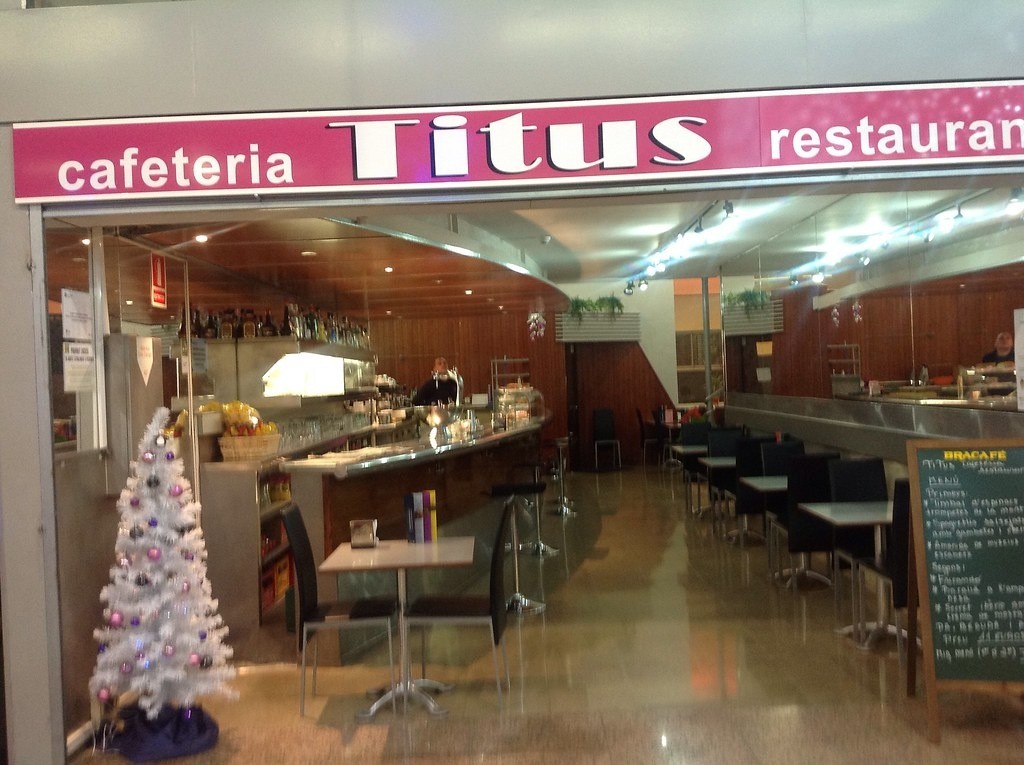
[933,376,953,385]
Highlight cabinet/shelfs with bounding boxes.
[236,335,379,408]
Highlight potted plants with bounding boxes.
[595,292,624,322]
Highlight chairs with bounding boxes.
[680,422,712,512]
[833,457,891,626]
[650,409,677,471]
[401,493,516,718]
[723,436,777,550]
[769,452,841,601]
[277,502,397,719]
[759,440,811,587]
[858,477,920,678]
[635,407,658,472]
[708,437,746,533]
[697,428,742,515]
[591,407,622,470]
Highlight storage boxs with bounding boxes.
[185,411,223,436]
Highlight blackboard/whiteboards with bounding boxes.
[903,437,1023,696]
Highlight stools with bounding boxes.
[486,478,548,617]
[544,436,580,516]
[512,460,558,558]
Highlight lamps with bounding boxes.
[638,271,648,291]
[624,281,635,295]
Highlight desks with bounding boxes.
[645,418,681,476]
[696,456,765,551]
[798,501,925,655]
[317,535,476,726]
[739,476,832,593]
[671,444,725,521]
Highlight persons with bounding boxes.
[411,357,457,405]
[983,332,1016,395]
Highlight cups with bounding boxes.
[377,408,392,424]
[466,409,476,432]
[277,410,370,454]
[365,400,376,413]
[349,518,378,548]
[352,400,363,410]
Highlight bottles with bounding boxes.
[177,300,369,351]
[920,364,928,385]
[366,392,410,408]
[259,482,272,511]
[373,373,397,384]
